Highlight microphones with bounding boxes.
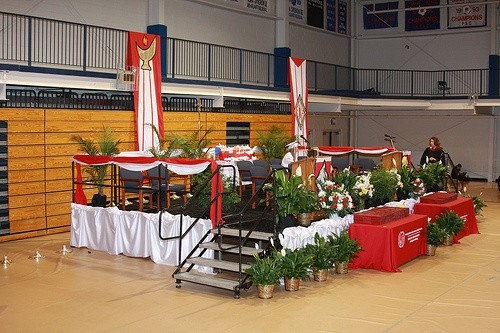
[385,134,395,138]
[300,135,310,144]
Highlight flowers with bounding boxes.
[318,156,449,215]
[458,188,487,216]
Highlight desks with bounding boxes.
[347,215,428,272]
[414,198,480,244]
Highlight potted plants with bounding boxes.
[248,229,362,299]
[273,172,320,227]
[426,222,443,255]
[74,129,121,208]
[433,209,464,248]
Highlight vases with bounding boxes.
[363,198,376,209]
[376,196,390,205]
[396,188,409,201]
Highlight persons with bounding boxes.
[451,164,469,180]
[420,137,445,168]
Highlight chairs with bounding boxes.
[116,143,382,209]
[438,81,450,94]
